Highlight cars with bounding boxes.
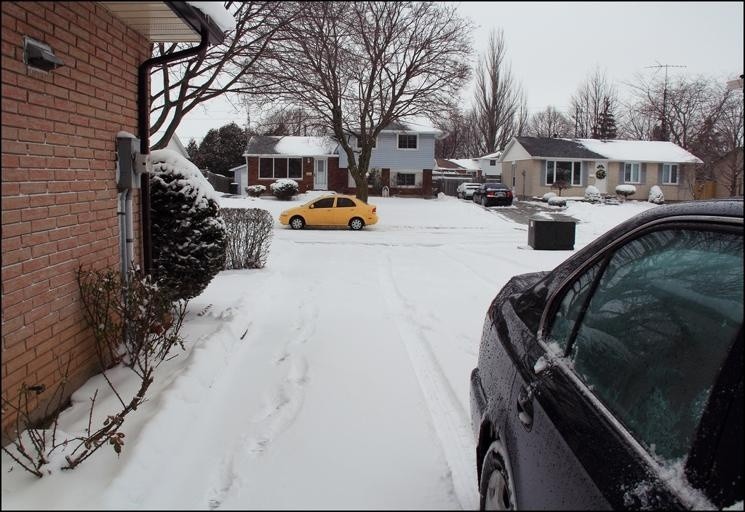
[457,181,514,207]
[470,196,745,511]
[278,193,379,230]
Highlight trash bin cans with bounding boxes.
[529,214,579,249]
[230,183,238,194]
